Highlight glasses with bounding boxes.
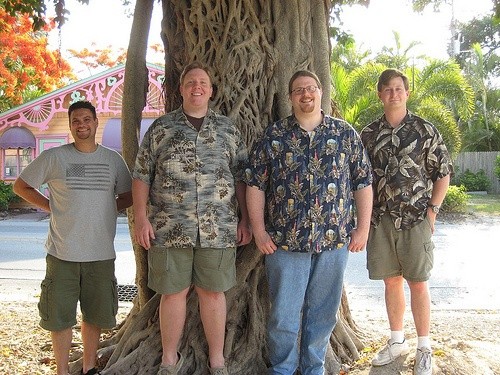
[290,85,319,96]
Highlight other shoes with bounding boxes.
[206,357,230,375]
[156,351,185,375]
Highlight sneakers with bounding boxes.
[414,347,432,375]
[371,338,409,366]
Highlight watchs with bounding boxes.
[428,204,439,214]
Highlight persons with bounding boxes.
[359,69,449,375]
[132,62,251,375]
[15,101,132,375]
[246,71,375,374]
